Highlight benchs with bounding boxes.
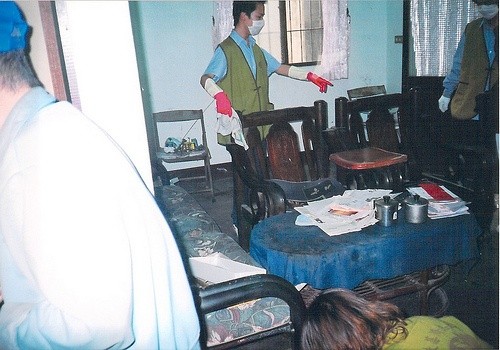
[149,185,306,350]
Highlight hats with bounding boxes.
[0,0,31,52]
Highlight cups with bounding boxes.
[375,196,399,227]
[402,195,429,224]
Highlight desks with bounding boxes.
[332,87,424,188]
[328,144,407,196]
[245,183,483,311]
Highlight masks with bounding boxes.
[243,14,265,36]
[477,4,498,20]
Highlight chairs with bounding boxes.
[153,109,216,205]
[231,100,341,251]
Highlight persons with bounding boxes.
[0,0,202,350]
[201,0,334,236]
[301,286,492,350]
[437,0,499,208]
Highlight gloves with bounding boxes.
[439,95,450,112]
[214,93,232,116]
[307,72,334,94]
